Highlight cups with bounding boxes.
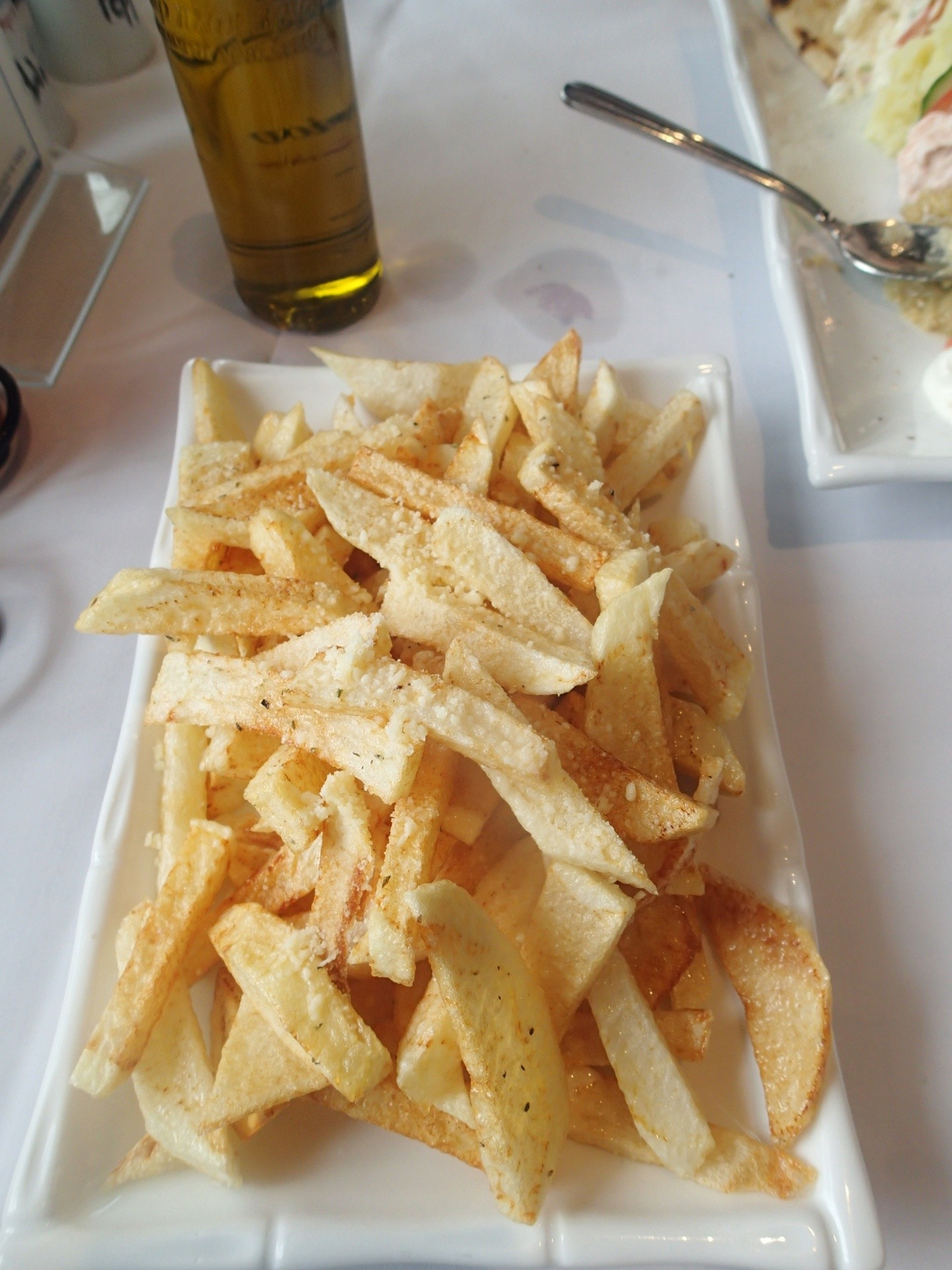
[147,0,385,335]
[28,0,158,84]
[0,0,78,161]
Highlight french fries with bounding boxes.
[72,330,834,1231]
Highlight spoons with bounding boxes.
[560,80,950,283]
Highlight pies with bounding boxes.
[764,0,853,86]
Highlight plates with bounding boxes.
[6,354,886,1270]
[711,0,951,488]
